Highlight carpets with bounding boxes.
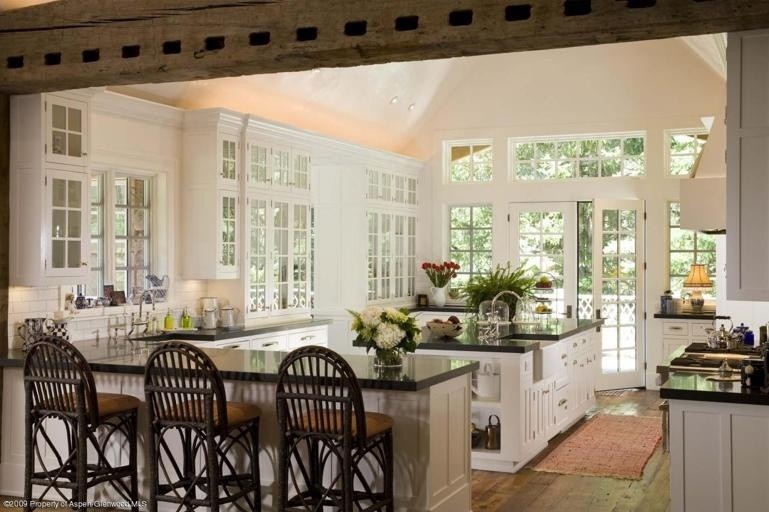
[524,412,662,481]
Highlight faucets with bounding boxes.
[487,290,526,338]
[129,290,156,338]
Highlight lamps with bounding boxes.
[684,265,712,314]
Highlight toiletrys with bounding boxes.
[182,305,193,329]
[165,307,175,329]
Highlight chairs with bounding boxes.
[23,335,140,512]
[144,341,262,512]
[275,345,395,512]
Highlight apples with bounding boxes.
[541,276,548,282]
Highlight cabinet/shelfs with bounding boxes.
[407,306,477,324]
[654,314,715,360]
[10,88,98,286]
[725,28,769,302]
[183,107,246,280]
[117,315,332,352]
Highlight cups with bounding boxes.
[75,293,86,310]
[96,300,103,307]
[86,298,94,308]
[68,304,77,311]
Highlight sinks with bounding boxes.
[495,339,561,380]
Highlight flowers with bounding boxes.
[422,261,460,288]
[344,305,423,349]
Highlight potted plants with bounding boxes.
[459,264,532,321]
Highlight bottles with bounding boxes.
[164,313,174,331]
[472,423,483,449]
[181,311,192,330]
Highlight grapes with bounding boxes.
[536,281,552,289]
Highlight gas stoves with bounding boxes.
[656,342,768,376]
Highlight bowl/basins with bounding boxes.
[428,320,466,338]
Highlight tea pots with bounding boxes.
[729,322,753,351]
[98,297,113,307]
[485,414,499,451]
[201,307,217,329]
[219,305,239,328]
[471,363,500,401]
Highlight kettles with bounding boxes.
[705,316,734,350]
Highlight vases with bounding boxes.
[374,349,403,367]
[429,286,446,307]
